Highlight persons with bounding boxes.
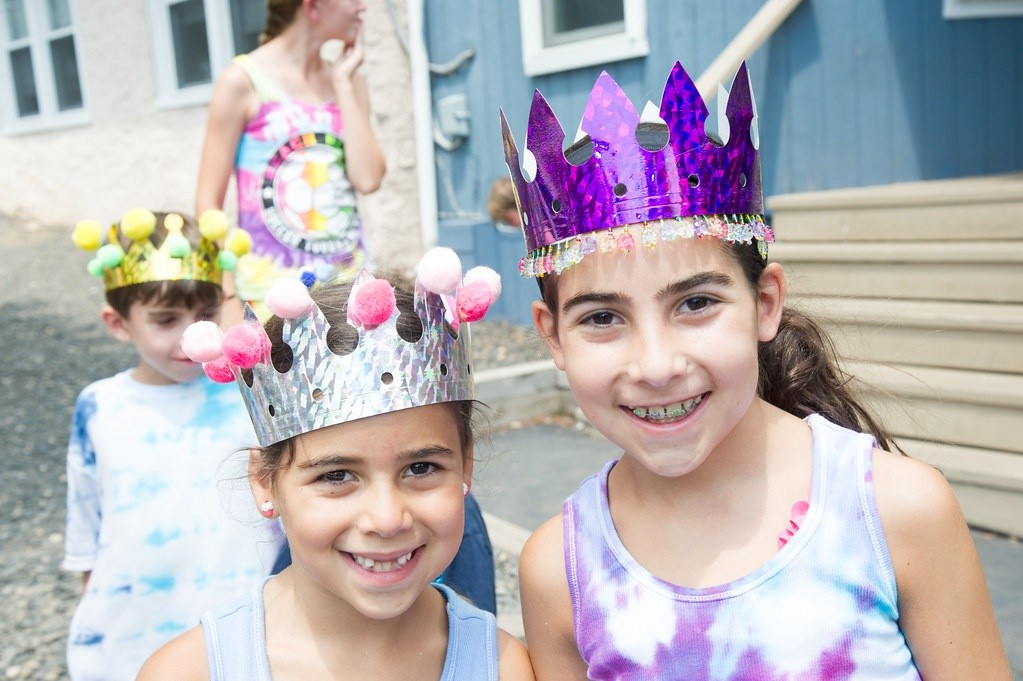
[490,182,526,228]
[198,0,386,289]
[273,494,496,623]
[134,246,537,681]
[498,60,1010,681]
[59,209,287,681]
